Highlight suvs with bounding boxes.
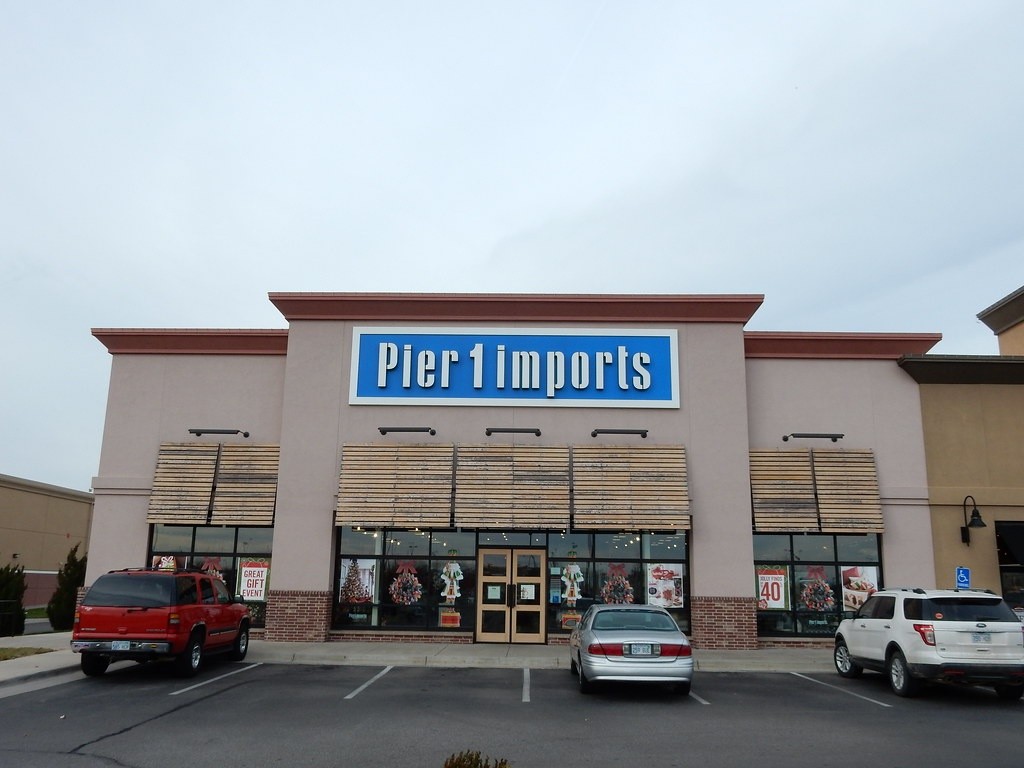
[834,585,1024,697]
[69,568,256,675]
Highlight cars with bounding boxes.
[570,603,692,696]
[555,597,603,626]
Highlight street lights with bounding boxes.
[961,495,988,547]
